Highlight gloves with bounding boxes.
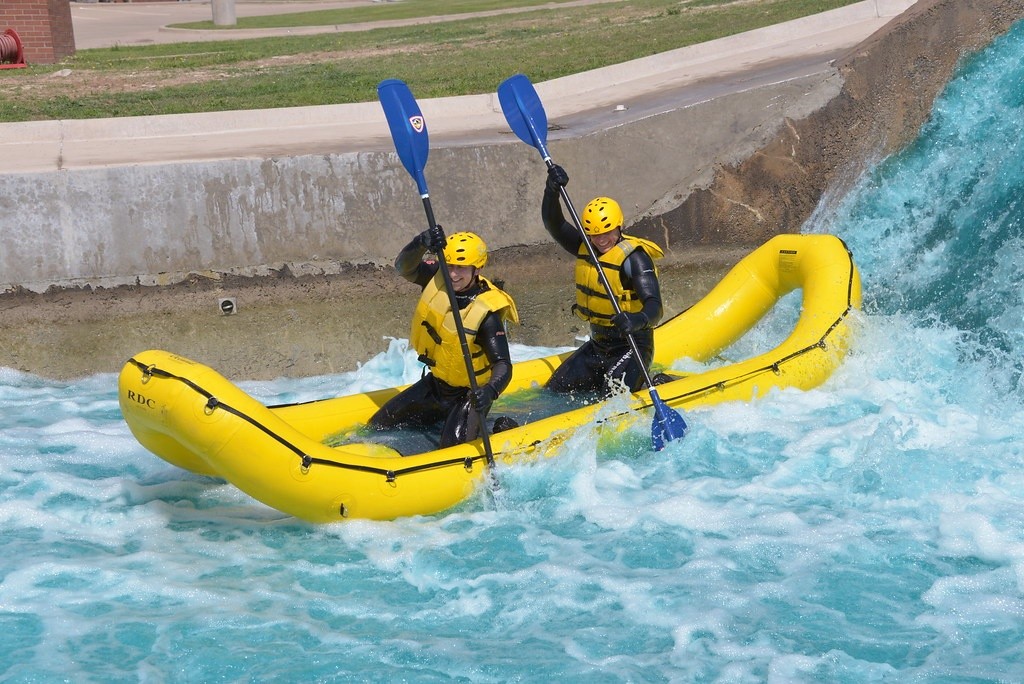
[610,311,650,340]
[418,224,447,253]
[473,383,498,417]
[545,164,569,197]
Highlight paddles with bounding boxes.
[497,75,687,452]
[378,79,499,494]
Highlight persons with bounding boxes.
[541,165,673,400]
[368,224,519,452]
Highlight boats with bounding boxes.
[119,223,862,524]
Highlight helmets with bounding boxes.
[434,231,487,268]
[582,196,624,235]
